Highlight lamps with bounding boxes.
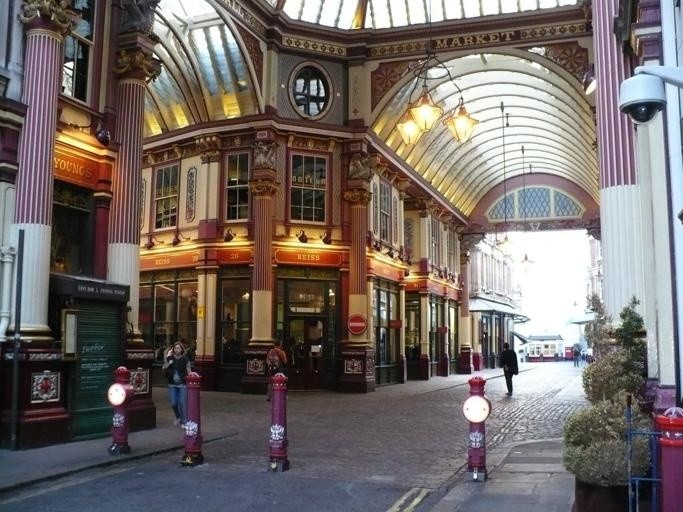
[395,0,480,147]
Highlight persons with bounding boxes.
[498,342,517,397]
[160,341,191,427]
[265,338,288,401]
[573,348,581,367]
[580,352,586,366]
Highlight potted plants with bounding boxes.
[562,389,652,512]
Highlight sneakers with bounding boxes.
[174,418,184,428]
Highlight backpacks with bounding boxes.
[269,350,281,370]
